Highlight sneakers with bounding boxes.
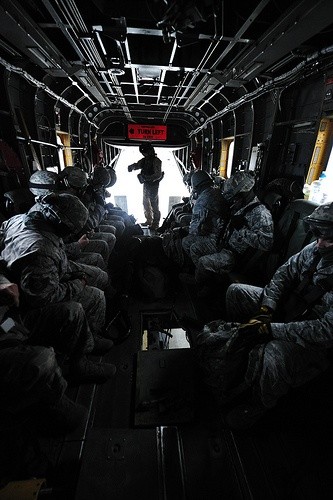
[93,336,114,352]
[84,363,116,378]
[59,393,88,426]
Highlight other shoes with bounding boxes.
[148,226,158,230]
[140,222,151,226]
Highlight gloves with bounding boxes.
[249,306,270,336]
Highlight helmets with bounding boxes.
[222,170,256,200]
[37,193,88,236]
[93,165,116,188]
[60,166,88,190]
[303,202,332,241]
[191,169,214,190]
[28,170,64,196]
[138,142,152,153]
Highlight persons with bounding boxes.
[128,142,164,232]
[156,168,333,432]
[0,164,128,432]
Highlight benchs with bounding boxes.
[0,378,96,500]
[178,188,332,500]
